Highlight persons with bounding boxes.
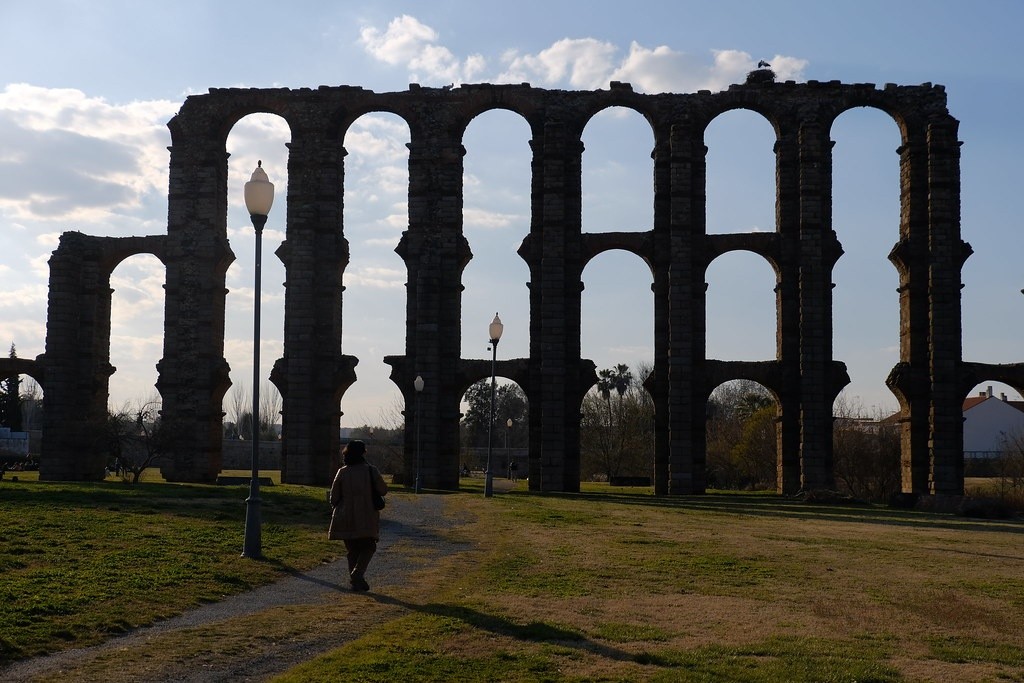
[461,459,517,483]
[328,440,388,589]
[1,453,120,476]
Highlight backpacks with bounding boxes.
[511,463,517,470]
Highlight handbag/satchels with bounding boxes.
[369,466,385,510]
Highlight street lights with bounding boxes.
[507,418,512,481]
[243,159,275,560]
[414,372,425,494]
[484,311,504,504]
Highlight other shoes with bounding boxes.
[350,568,369,590]
